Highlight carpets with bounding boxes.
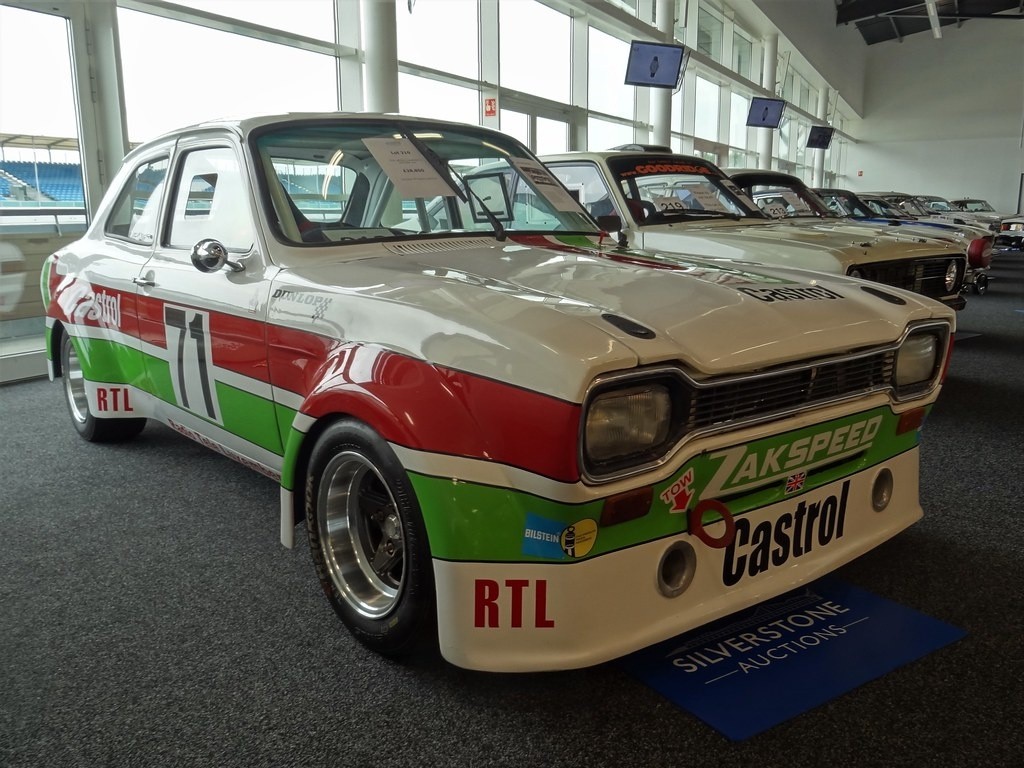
[633,586,960,742]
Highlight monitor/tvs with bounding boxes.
[806,126,833,149]
[623,40,686,89]
[746,97,785,128]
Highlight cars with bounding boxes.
[36,110,961,676]
[555,168,1024,294]
[392,149,969,317]
